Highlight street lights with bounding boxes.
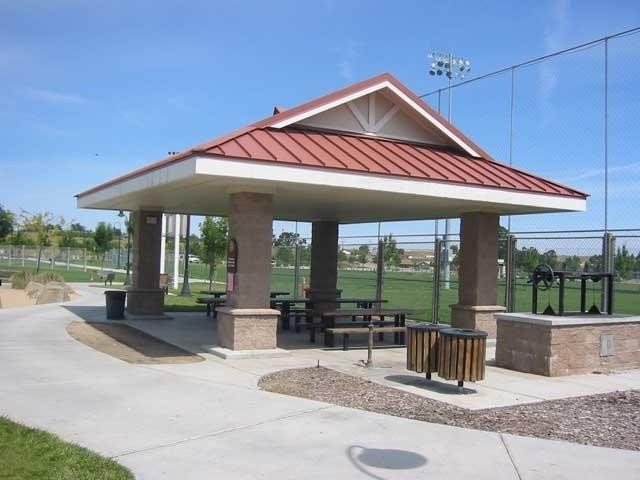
[117,211,126,268]
[427,49,472,292]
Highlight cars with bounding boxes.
[49,255,63,261]
[178,254,201,264]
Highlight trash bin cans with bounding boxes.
[407,324,488,393]
[104,291,126,320]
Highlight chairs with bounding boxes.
[104,273,115,286]
[159,274,168,295]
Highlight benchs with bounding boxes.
[196,289,408,350]
